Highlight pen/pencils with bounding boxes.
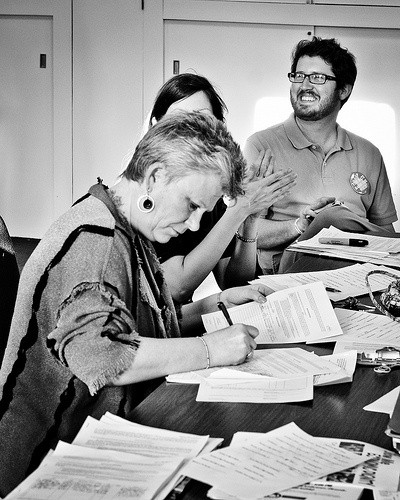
[217,300,234,326]
[324,285,341,293]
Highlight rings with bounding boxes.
[247,351,252,357]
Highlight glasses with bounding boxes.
[288,71,336,84]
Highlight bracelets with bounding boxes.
[218,292,222,301]
[294,218,303,235]
[235,230,259,242]
[196,336,210,369]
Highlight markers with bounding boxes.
[318,236,370,247]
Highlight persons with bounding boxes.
[0,109,275,500]
[242,36,398,275]
[150,73,297,304]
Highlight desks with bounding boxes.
[123,231,400,500]
